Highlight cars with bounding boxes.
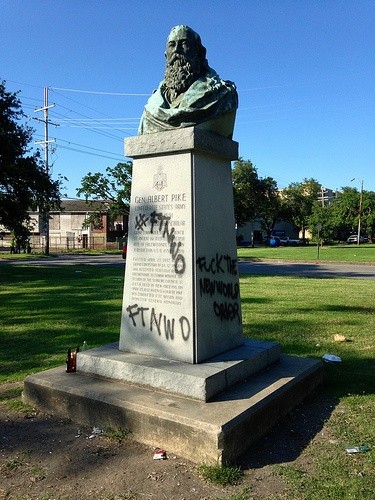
[347,234,365,245]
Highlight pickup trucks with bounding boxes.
[280,236,303,246]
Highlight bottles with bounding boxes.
[82,340,88,352]
[65,348,73,373]
[73,346,79,372]
